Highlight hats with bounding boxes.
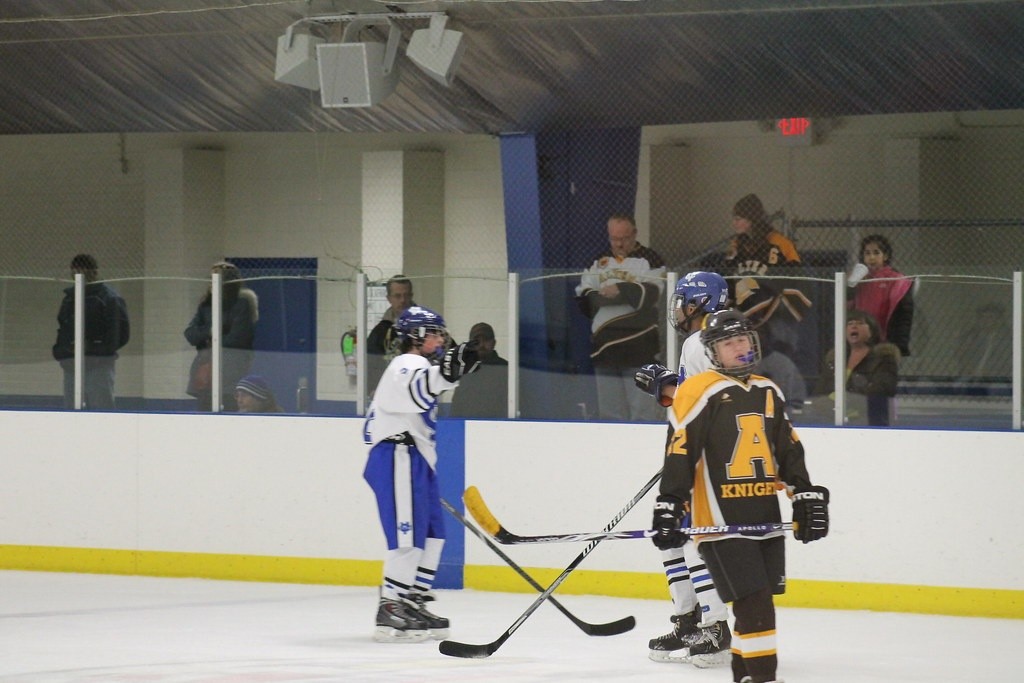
[734,193,765,222]
[235,373,272,401]
[469,323,493,341]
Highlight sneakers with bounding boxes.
[689,619,731,668]
[375,598,450,643]
[648,604,702,663]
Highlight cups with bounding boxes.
[847,263,869,288]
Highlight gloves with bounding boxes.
[651,495,688,550]
[791,486,829,544]
[634,363,679,407]
[441,341,480,383]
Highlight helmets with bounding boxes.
[397,307,452,358]
[700,310,762,375]
[668,270,728,333]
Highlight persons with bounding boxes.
[846,235,919,355]
[236,375,283,413]
[575,215,665,423]
[53,254,129,413]
[184,261,258,411]
[812,308,901,429]
[722,193,801,407]
[651,310,830,683]
[366,274,457,401]
[449,321,531,419]
[361,307,482,644]
[635,270,733,668]
[752,315,806,416]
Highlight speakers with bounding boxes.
[315,42,402,108]
[404,28,465,87]
[274,34,326,91]
